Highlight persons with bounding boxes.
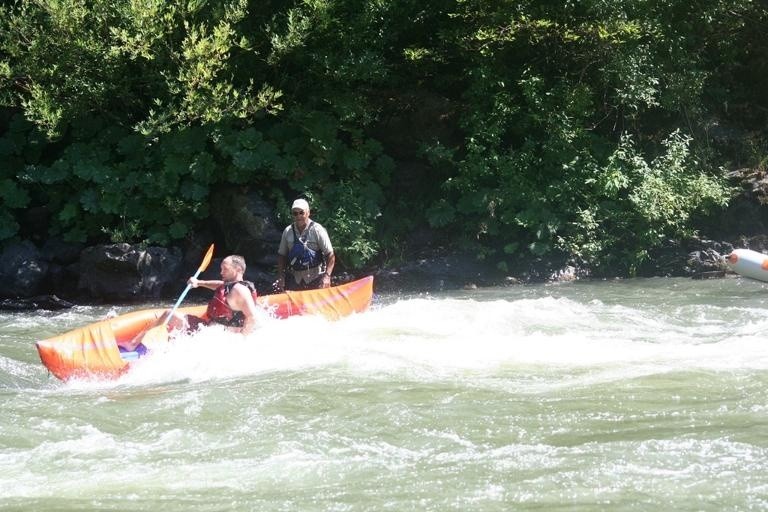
[274,198,337,294]
[117,253,261,353]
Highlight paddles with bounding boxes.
[141,244,214,348]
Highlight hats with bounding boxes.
[292,199,309,210]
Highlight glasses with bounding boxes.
[293,211,305,216]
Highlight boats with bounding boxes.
[35,273,373,386]
[723,247,767,289]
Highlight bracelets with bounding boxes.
[324,271,332,277]
